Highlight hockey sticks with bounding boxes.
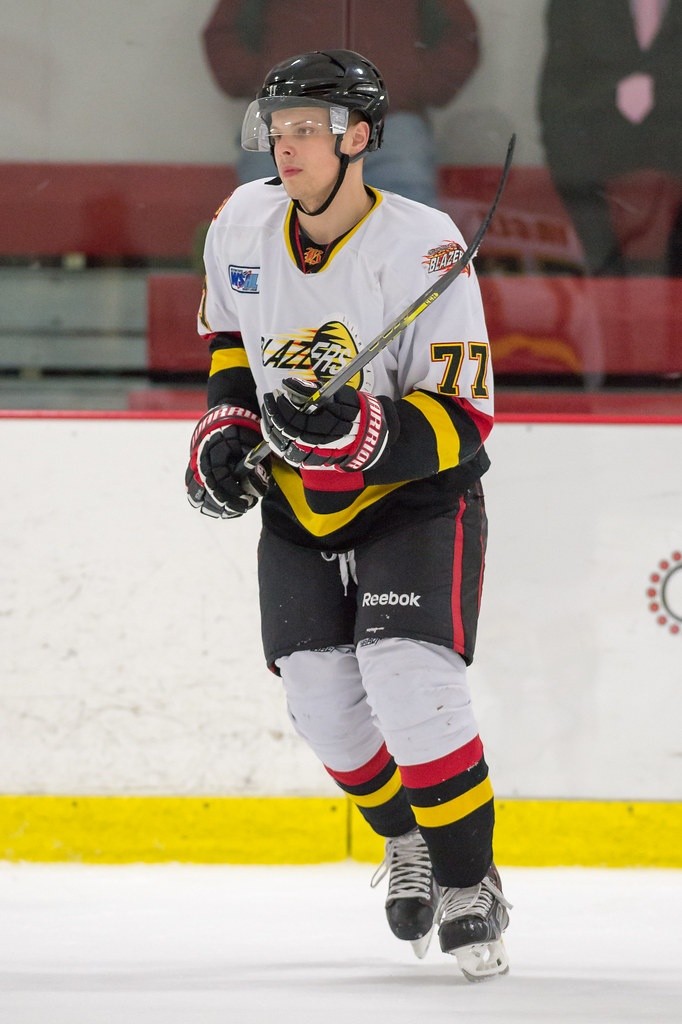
[222,129,520,488]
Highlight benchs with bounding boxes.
[149,274,682,380]
[0,160,682,274]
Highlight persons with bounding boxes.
[198,0,485,209]
[534,0,682,277]
[184,47,514,983]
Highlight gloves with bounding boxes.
[258,379,387,476]
[180,402,270,518]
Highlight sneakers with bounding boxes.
[370,827,444,961]
[433,860,514,984]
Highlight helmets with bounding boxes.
[258,49,387,149]
